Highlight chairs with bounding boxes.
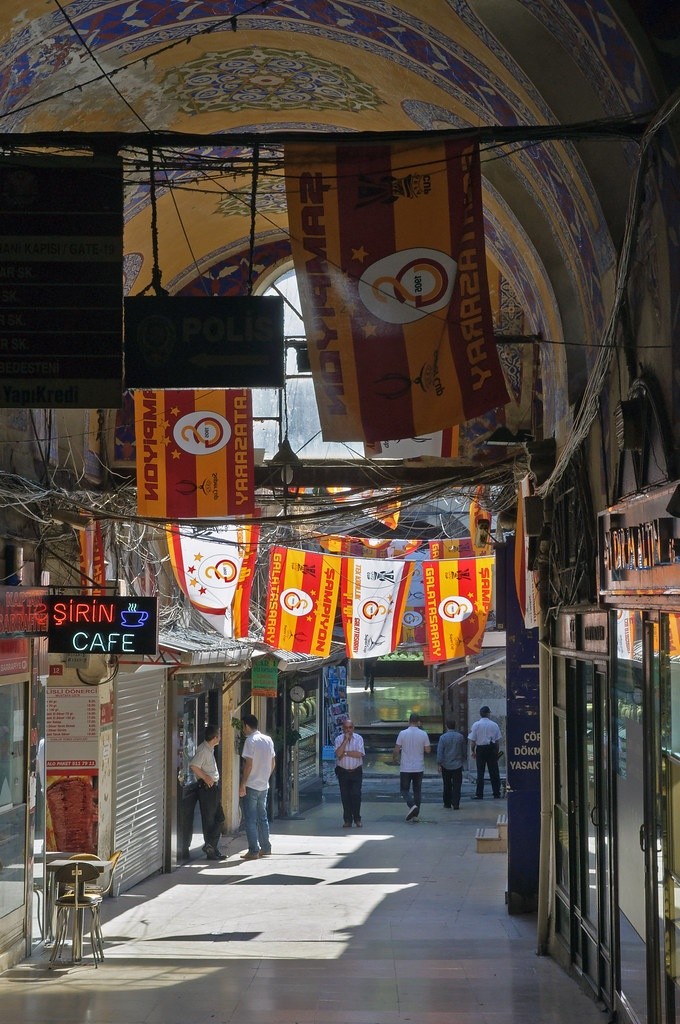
[61,850,122,964]
[47,861,103,970]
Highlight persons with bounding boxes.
[468,706,503,800]
[362,657,378,693]
[435,718,468,811]
[392,713,431,823]
[333,719,367,827]
[239,713,277,860]
[191,724,230,860]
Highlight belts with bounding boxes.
[341,769,356,773]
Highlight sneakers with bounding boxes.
[405,805,421,822]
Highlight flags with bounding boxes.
[263,541,342,659]
[616,607,637,662]
[513,472,543,630]
[360,423,460,461]
[75,516,107,600]
[423,557,499,666]
[319,484,404,530]
[388,547,428,649]
[468,481,493,556]
[652,611,680,661]
[316,532,424,559]
[426,534,497,561]
[132,386,254,520]
[336,555,415,661]
[277,139,511,443]
[279,482,305,499]
[163,508,262,642]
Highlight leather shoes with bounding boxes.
[258,850,271,855]
[240,851,260,859]
[471,795,483,799]
[354,821,363,827]
[444,804,451,808]
[454,806,460,809]
[343,821,352,827]
[202,843,228,860]
[494,794,500,798]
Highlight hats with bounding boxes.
[480,706,492,713]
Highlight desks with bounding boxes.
[46,860,113,962]
